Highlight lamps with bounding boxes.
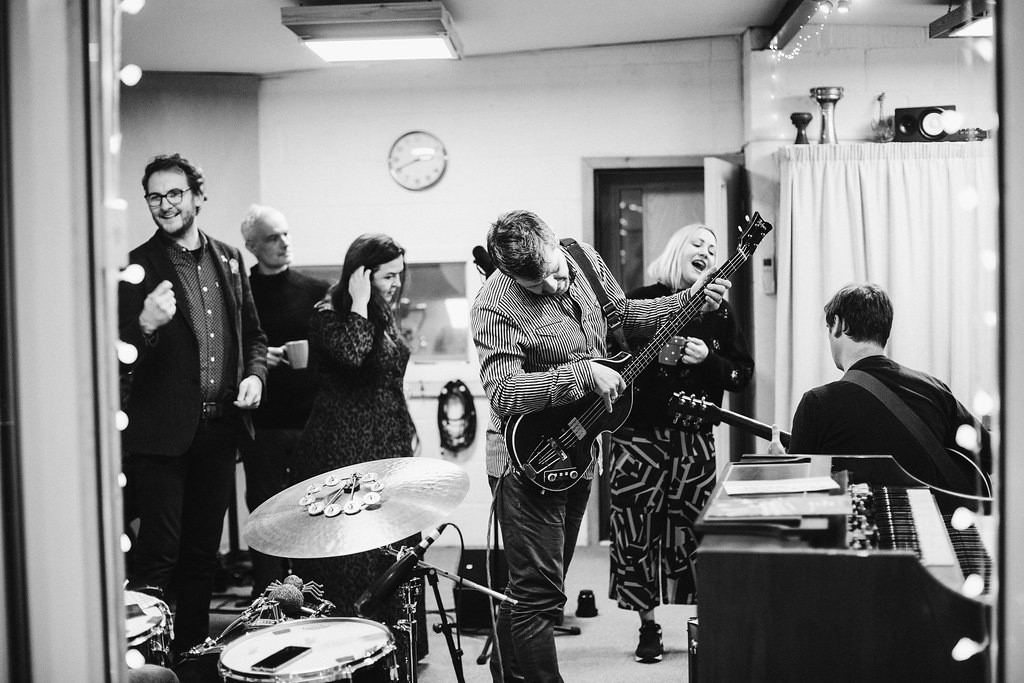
[929,0,996,39]
[280,0,463,62]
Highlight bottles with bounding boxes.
[766,424,785,455]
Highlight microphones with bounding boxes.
[354,523,448,614]
[472,246,497,276]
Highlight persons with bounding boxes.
[118,151,270,683]
[767,282,980,513]
[601,223,756,663]
[306,229,430,666]
[239,201,333,594]
[471,209,734,683]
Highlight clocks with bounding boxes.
[388,131,447,191]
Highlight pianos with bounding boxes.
[694,454,995,683]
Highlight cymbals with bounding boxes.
[242,456,472,559]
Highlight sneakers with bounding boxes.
[635,619,664,661]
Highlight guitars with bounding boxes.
[667,391,791,449]
[503,211,773,493]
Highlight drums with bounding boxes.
[124,589,174,668]
[217,617,399,683]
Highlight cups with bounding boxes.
[658,335,692,365]
[278,340,308,370]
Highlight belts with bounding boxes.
[201,403,224,420]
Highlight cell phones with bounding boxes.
[251,646,311,672]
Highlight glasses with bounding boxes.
[144,187,191,206]
